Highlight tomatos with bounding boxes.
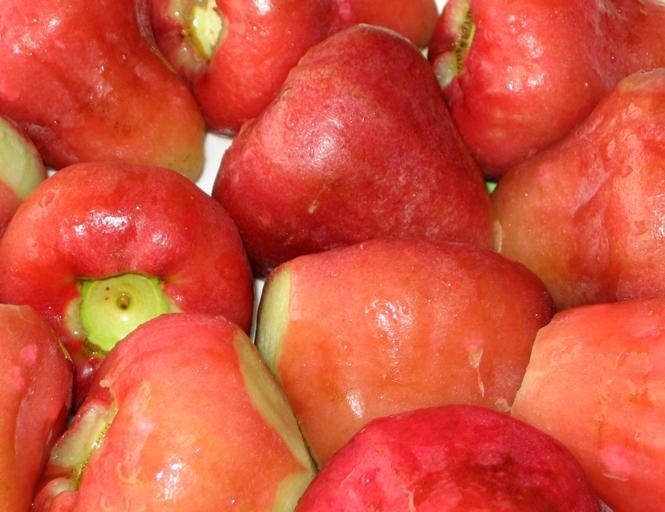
[0,0,665,512]
